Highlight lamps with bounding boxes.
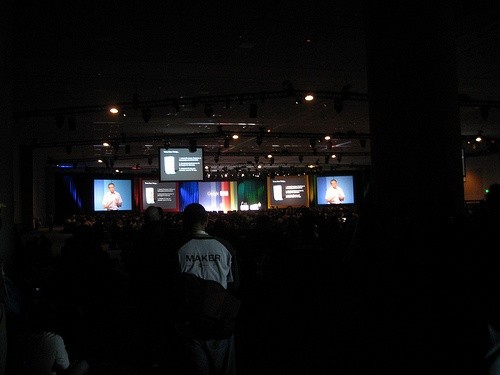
[48,91,367,177]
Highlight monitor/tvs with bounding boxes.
[92,177,135,212]
[157,145,205,183]
[316,174,356,206]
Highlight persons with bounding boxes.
[14,324,91,375]
[175,202,238,375]
[47,212,55,232]
[102,182,123,211]
[63,204,345,250]
[325,178,346,204]
